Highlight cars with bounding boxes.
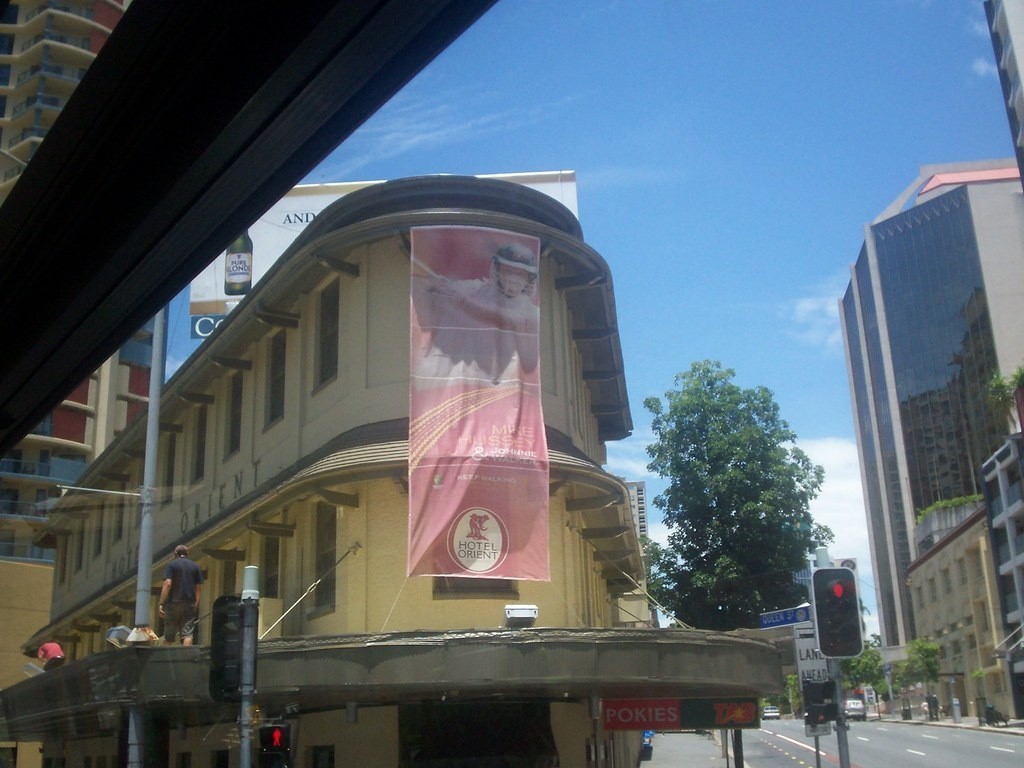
[844,700,867,721]
[762,706,780,720]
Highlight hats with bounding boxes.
[174,545,188,556]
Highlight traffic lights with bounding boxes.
[804,679,839,725]
[257,721,289,768]
[814,567,865,659]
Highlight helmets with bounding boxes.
[494,242,539,276]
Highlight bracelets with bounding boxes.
[159,602,164,606]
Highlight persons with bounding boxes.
[38,642,65,671]
[921,691,939,721]
[412,242,539,380]
[158,545,204,646]
